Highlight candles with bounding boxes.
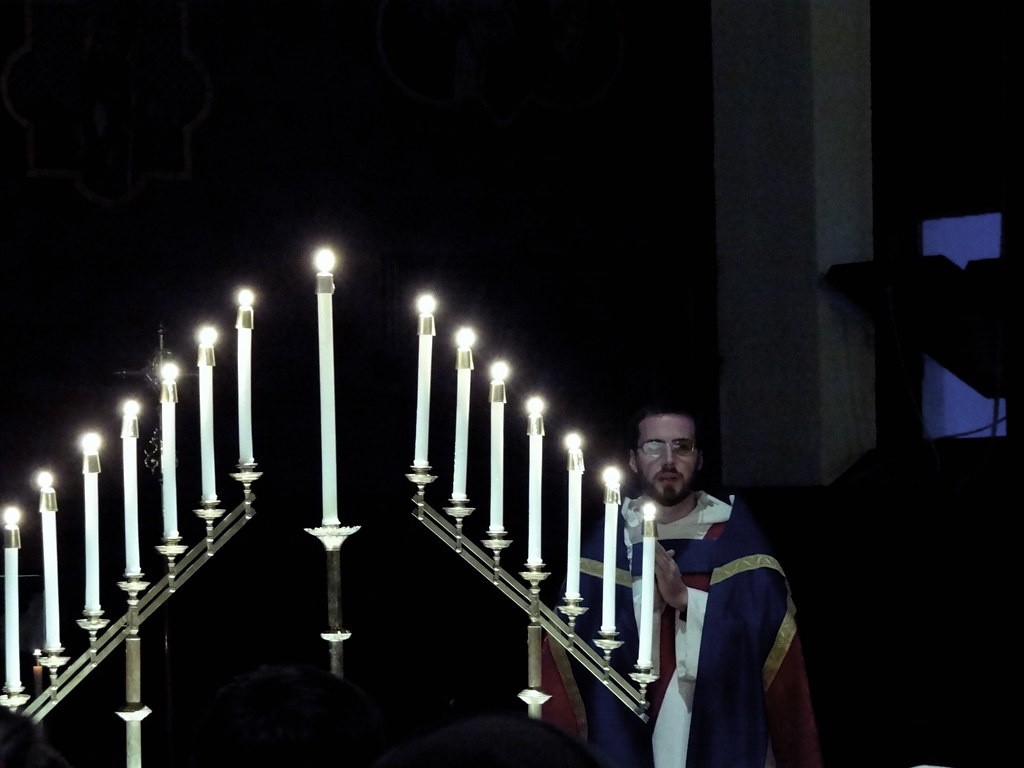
[310,246,340,525]
[118,400,141,574]
[451,325,475,503]
[600,464,615,636]
[486,356,511,531]
[561,434,584,599]
[636,500,657,671]
[199,327,219,507]
[38,473,60,650]
[236,287,256,465]
[525,394,546,570]
[156,359,181,540]
[0,506,24,689]
[411,289,436,469]
[81,432,101,610]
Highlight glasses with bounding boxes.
[632,438,697,458]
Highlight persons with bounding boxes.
[194,668,599,768]
[543,407,822,768]
